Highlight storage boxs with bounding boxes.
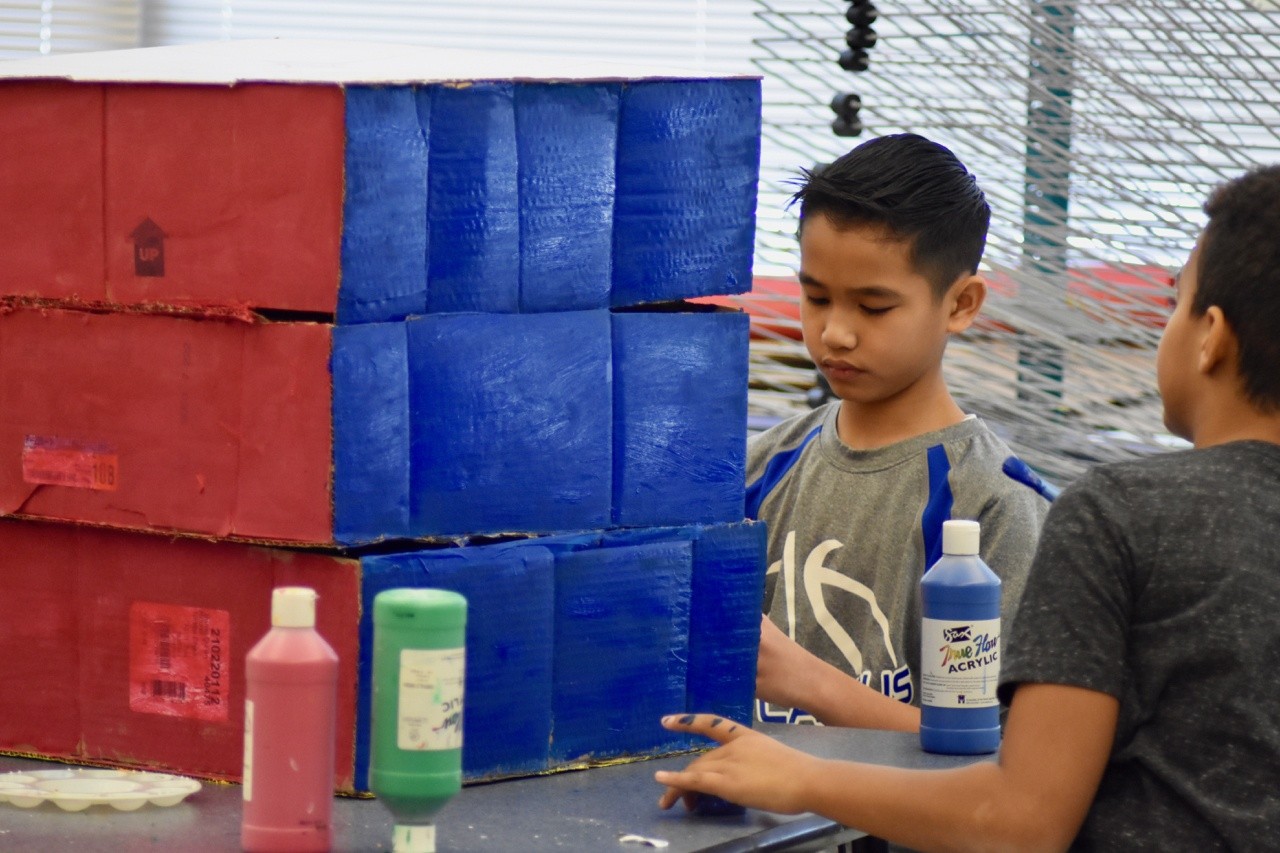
[0,35,772,806]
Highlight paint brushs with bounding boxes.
[1002,457,1059,502]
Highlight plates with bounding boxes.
[0,768,201,811]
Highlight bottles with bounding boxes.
[368,587,468,853]
[239,587,339,853]
[919,519,1001,756]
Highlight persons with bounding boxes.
[751,131,1054,731]
[655,166,1279,852]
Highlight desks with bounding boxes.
[0,719,1004,853]
